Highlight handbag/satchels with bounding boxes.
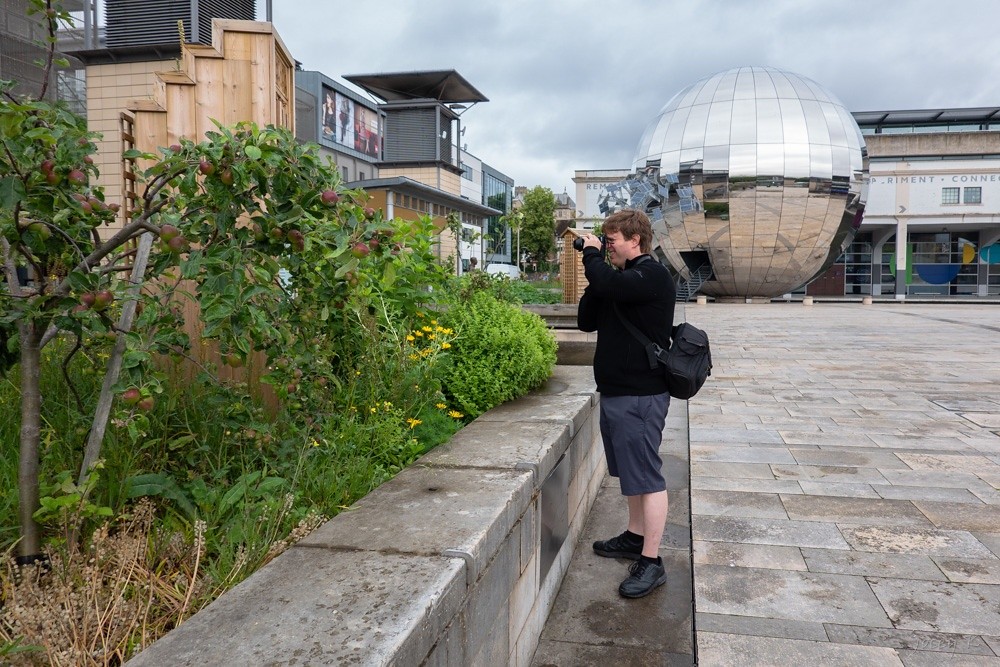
[665,322,713,399]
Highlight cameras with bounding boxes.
[574,234,606,260]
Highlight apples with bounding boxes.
[0,117,478,449]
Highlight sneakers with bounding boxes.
[619,556,667,598]
[593,531,643,560]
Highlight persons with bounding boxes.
[323,90,336,141]
[339,99,350,141]
[469,256,478,271]
[577,208,676,598]
[360,107,371,154]
